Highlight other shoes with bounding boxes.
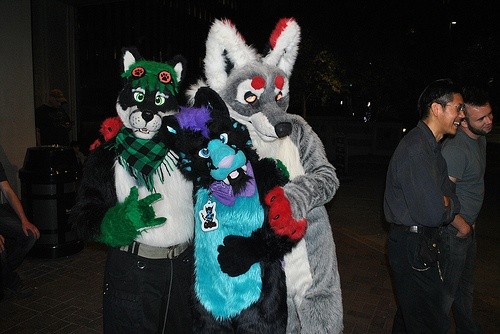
[0,283,32,298]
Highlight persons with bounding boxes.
[0,162,40,253]
[381,79,492,334]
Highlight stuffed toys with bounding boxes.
[69,18,344,334]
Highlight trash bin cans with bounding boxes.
[18,145,84,259]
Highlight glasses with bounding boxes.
[441,104,466,112]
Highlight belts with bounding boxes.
[391,224,434,235]
